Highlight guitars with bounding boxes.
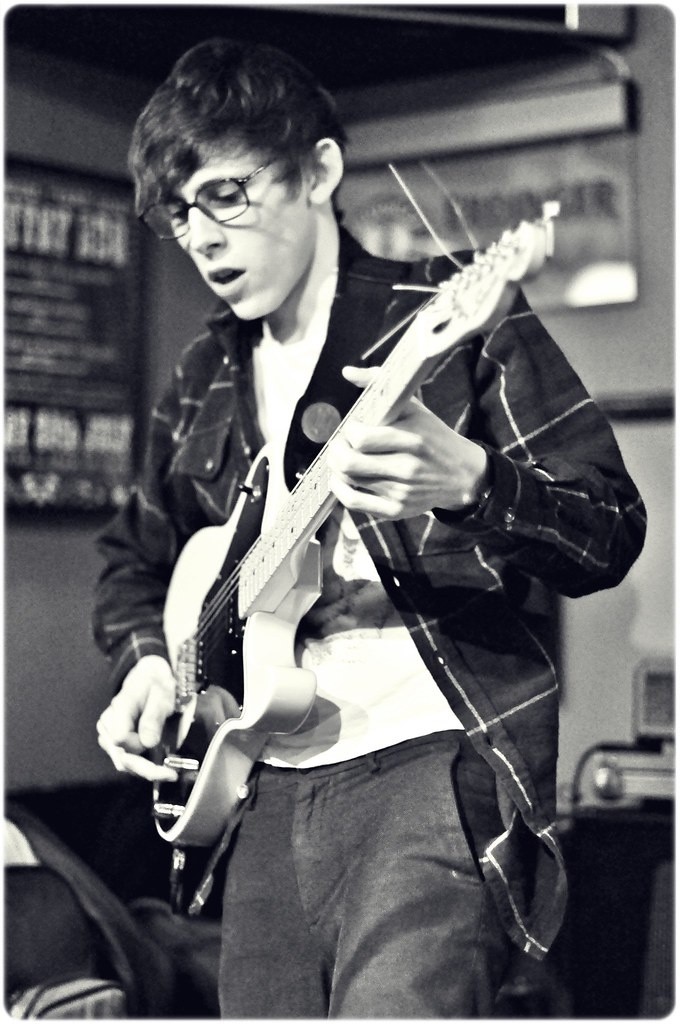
[152,197,568,854]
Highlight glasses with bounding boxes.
[137,134,306,240]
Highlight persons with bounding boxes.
[97,39,648,1019]
